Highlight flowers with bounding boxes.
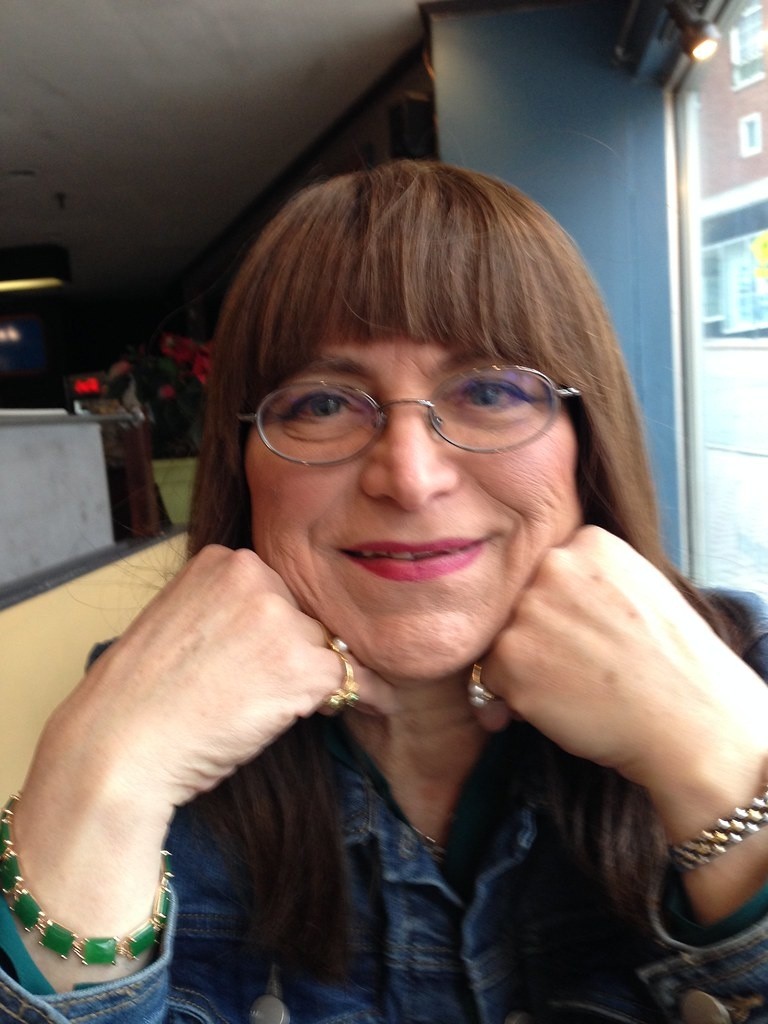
[140,321,223,459]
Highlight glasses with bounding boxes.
[236,366,582,466]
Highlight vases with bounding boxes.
[151,457,197,524]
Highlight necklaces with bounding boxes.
[408,822,437,845]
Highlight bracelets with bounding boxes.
[669,790,767,872]
[1,794,173,967]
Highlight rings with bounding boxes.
[314,620,360,709]
[468,660,495,708]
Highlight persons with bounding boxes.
[0,164,768,1024]
